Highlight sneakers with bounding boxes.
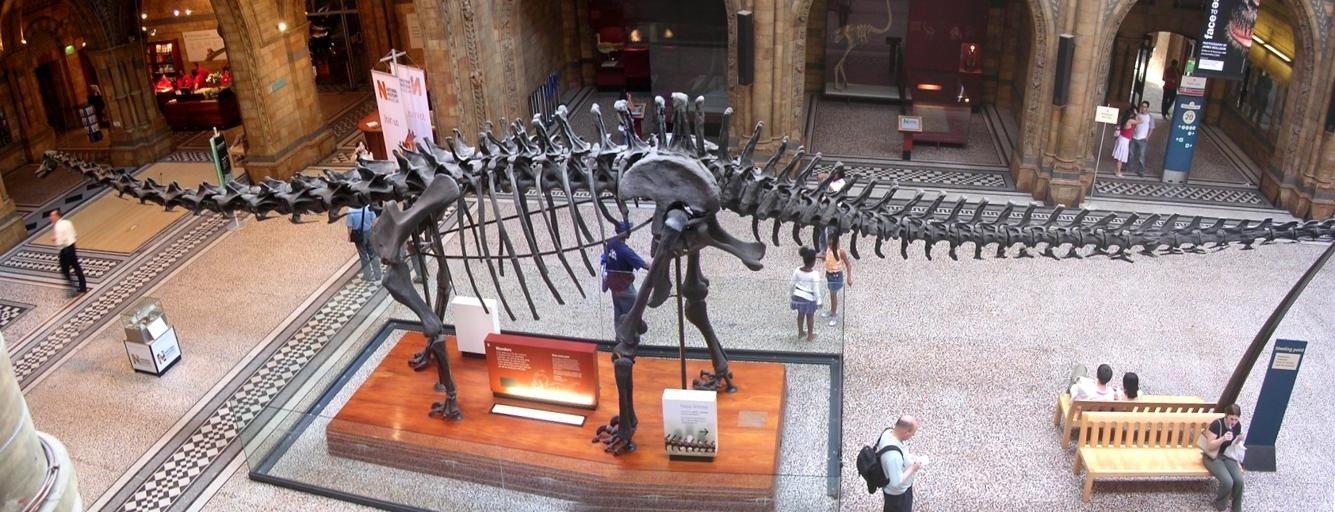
[808,332,819,340]
[374,270,382,280]
[1136,170,1144,177]
[77,288,87,293]
[1162,111,1169,120]
[412,277,424,284]
[364,270,373,280]
[798,329,808,338]
[1114,169,1124,179]
[822,308,838,326]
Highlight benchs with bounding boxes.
[1053,392,1246,504]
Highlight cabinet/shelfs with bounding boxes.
[143,39,185,90]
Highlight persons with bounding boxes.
[617,87,636,146]
[351,138,374,163]
[788,246,823,340]
[50,210,87,295]
[1111,100,1155,179]
[600,220,648,343]
[346,204,382,281]
[1114,372,1144,400]
[1161,58,1182,120]
[1247,70,1272,128]
[1202,404,1245,511]
[154,61,235,95]
[877,415,922,512]
[1066,363,1114,411]
[815,233,854,327]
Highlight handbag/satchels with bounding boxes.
[1223,437,1247,464]
[349,229,364,243]
[1113,125,1121,138]
[1196,418,1222,460]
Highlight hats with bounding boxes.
[614,221,634,234]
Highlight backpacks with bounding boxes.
[856,427,904,494]
